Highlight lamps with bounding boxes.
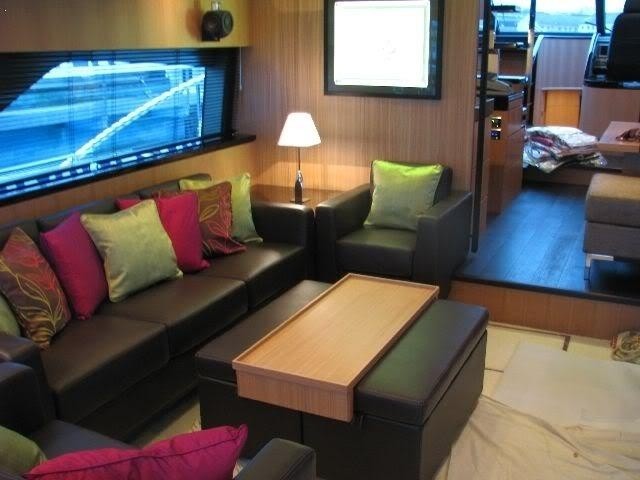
[277,112,322,204]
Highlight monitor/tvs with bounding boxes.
[323,1,444,98]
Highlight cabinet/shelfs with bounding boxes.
[487,105,525,215]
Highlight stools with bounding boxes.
[583,171,640,269]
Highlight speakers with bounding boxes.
[202,11,233,42]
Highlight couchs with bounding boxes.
[0,174,316,443]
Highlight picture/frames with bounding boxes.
[323,1,444,100]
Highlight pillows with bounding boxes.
[1,226,72,348]
[179,173,263,243]
[39,211,107,322]
[362,159,445,233]
[2,300,22,337]
[0,423,249,479]
[0,425,48,480]
[81,198,182,303]
[154,181,246,257]
[117,191,211,272]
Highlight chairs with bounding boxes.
[606,0,640,84]
[317,159,473,299]
[0,360,317,480]
